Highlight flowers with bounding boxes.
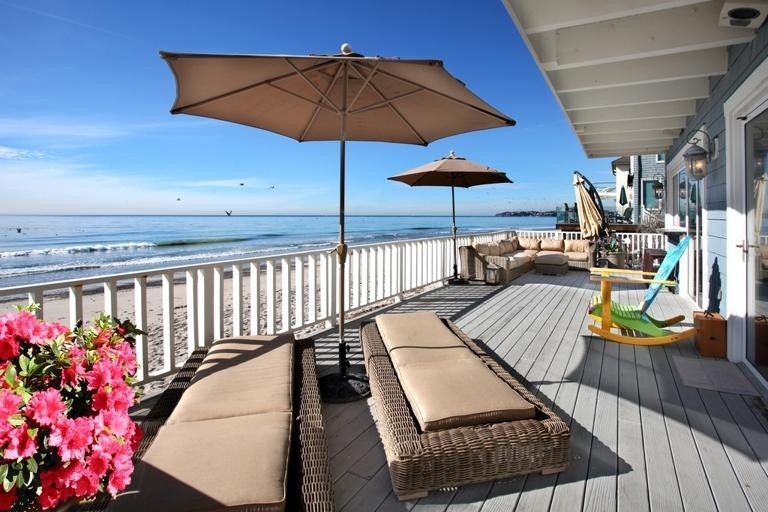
[1,303,148,511]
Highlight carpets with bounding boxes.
[674,355,762,397]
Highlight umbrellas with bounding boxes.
[385,149,515,277]
[155,39,515,375]
[571,170,604,240]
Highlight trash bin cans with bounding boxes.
[484,263,503,285]
[598,259,608,267]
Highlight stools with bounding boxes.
[534,249,570,275]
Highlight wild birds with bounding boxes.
[225,210,233,217]
[16,227,22,233]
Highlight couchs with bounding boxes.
[459,236,593,284]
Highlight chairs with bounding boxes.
[586,234,698,346]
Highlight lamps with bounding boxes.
[682,130,719,182]
[753,125,768,179]
[679,173,691,199]
[652,173,666,199]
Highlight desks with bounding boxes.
[643,249,675,294]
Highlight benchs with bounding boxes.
[64,334,336,512]
[359,311,573,502]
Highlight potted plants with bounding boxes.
[604,239,626,269]
